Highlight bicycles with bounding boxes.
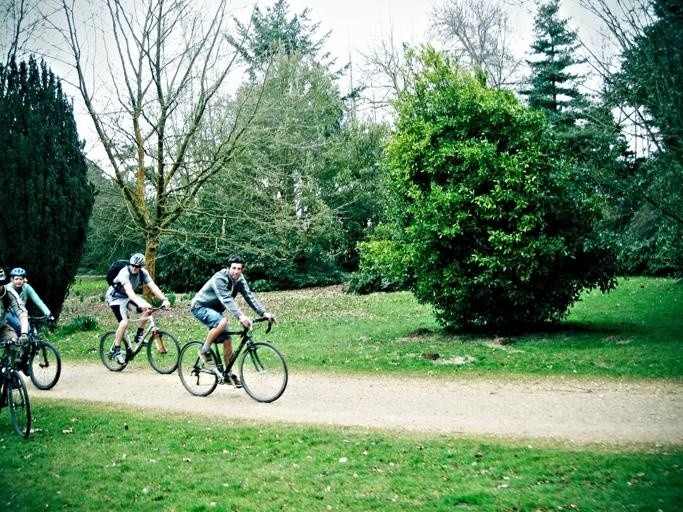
[0,314,63,428]
[176,315,289,403]
[99,303,180,374]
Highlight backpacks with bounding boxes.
[106,260,130,285]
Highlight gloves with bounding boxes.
[18,334,29,347]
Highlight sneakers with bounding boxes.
[134,335,148,345]
[23,362,31,376]
[226,374,243,388]
[111,354,127,366]
[197,347,215,367]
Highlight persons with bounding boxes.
[0,266,29,390]
[101,252,171,367]
[188,255,278,389]
[4,266,55,377]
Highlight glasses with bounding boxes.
[130,264,142,268]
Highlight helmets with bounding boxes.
[0,267,7,282]
[10,268,27,277]
[130,253,145,266]
